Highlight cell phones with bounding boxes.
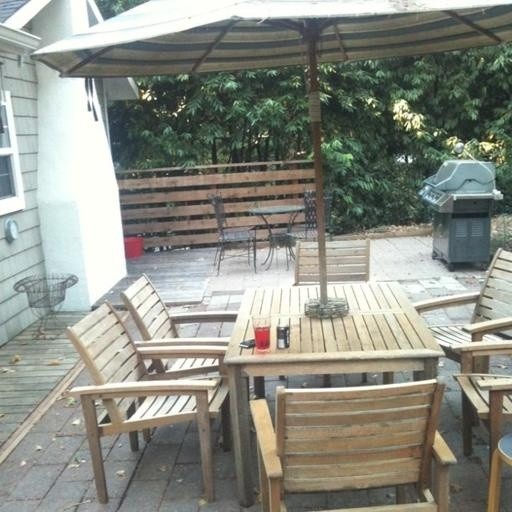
[239,340,255,349]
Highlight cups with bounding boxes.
[252,314,272,353]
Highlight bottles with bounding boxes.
[276,326,290,349]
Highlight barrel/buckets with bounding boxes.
[124,236,144,258]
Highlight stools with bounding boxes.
[484,431,512,511]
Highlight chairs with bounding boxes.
[292,237,373,286]
[283,191,336,242]
[414,246,511,481]
[117,271,239,450]
[250,378,462,512]
[210,191,258,274]
[60,299,230,504]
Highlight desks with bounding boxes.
[247,205,306,271]
[223,283,445,510]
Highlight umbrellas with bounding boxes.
[29,0,512,304]
